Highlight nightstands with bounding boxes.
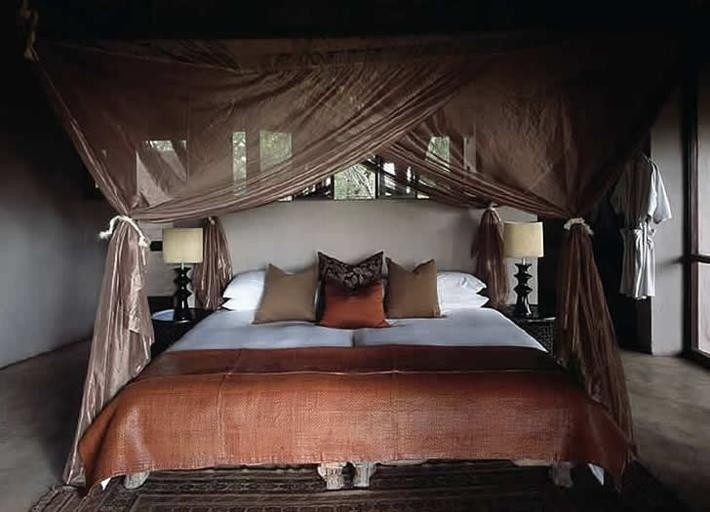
[495,301,558,357]
[151,306,213,354]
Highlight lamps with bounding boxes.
[159,222,209,325]
[502,218,550,316]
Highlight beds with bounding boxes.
[112,196,589,498]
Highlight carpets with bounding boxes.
[20,439,688,511]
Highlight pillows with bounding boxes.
[220,247,491,330]
